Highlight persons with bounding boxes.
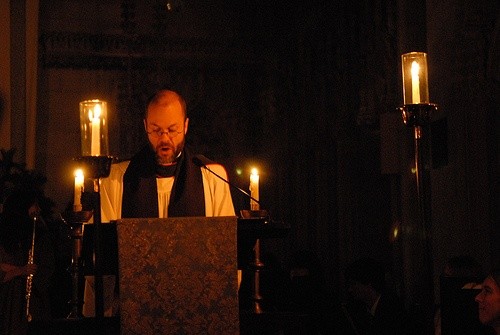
[272,251,335,319]
[0,192,96,335]
[82,88,243,300]
[343,253,406,335]
[475,270,500,333]
[433,251,469,287]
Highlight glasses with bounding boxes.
[145,125,184,137]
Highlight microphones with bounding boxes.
[192,156,270,209]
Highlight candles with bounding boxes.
[249,166,260,210]
[74,169,85,211]
[408,60,422,104]
[89,106,104,156]
